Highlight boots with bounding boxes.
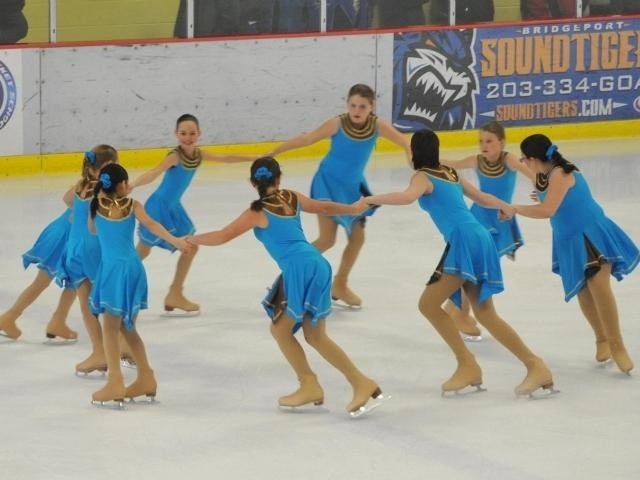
[347,368,382,412]
[47,313,78,339]
[442,301,480,336]
[597,337,633,373]
[0,307,21,337]
[76,336,157,401]
[165,286,201,311]
[441,357,482,391]
[515,359,554,395]
[279,375,323,406]
[332,276,362,305]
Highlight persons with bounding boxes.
[0,181,82,339]
[0,1,27,43]
[63,145,134,372]
[355,130,553,394]
[265,84,412,306]
[184,157,382,413]
[129,114,265,313]
[88,163,196,401]
[498,134,639,373]
[439,122,535,334]
